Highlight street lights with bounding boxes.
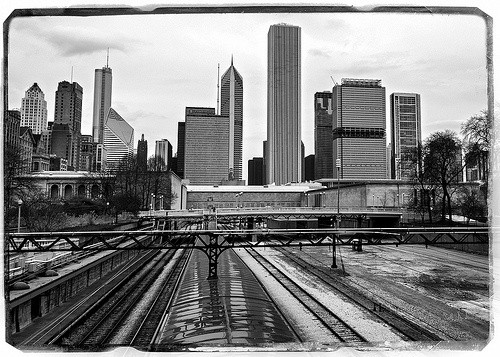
[334,158,342,242]
[17,198,23,235]
